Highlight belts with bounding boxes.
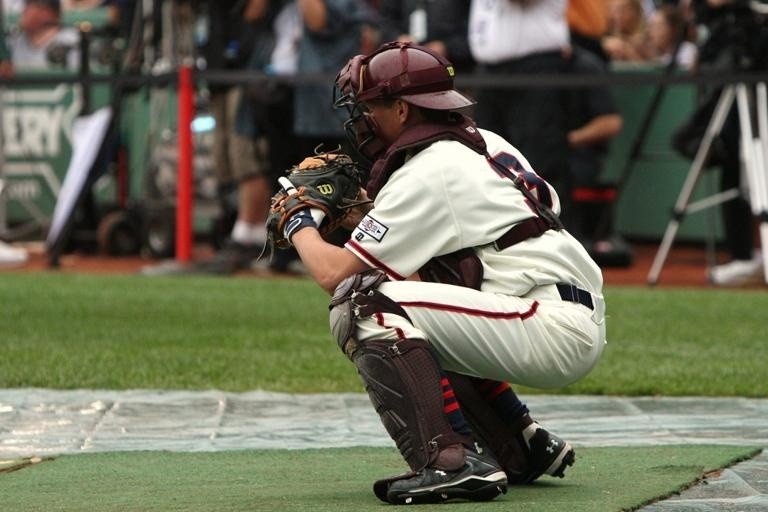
[556,283,594,310]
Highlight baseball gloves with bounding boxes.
[266,152,362,252]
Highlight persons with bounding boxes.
[254,41,608,507]
[0,0,767,288]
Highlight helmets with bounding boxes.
[334,42,478,164]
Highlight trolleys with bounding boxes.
[57,16,143,260]
[148,0,242,262]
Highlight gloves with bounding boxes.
[278,176,326,246]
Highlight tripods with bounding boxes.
[647,80,768,287]
[589,5,719,285]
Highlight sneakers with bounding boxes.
[517,428,575,483]
[201,236,270,274]
[708,257,764,285]
[388,447,508,504]
